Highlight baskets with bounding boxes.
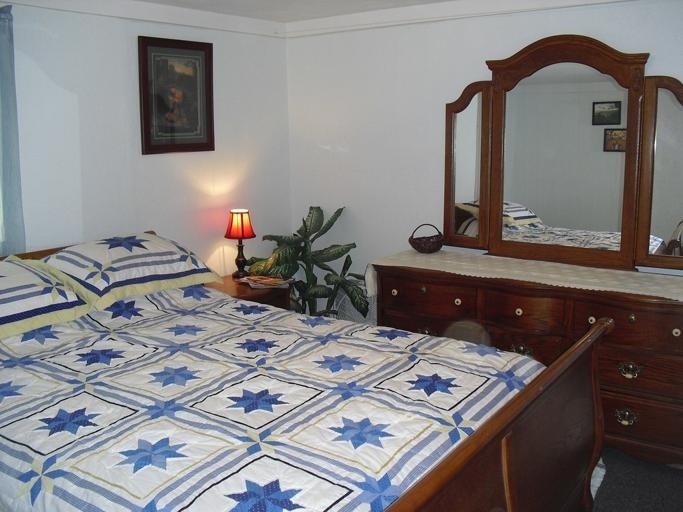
[408,223,443,254]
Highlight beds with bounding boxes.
[499,221,683,259]
[0,243,615,512]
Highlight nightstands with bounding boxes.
[207,275,295,310]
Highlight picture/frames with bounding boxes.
[138,35,216,155]
[603,128,627,152]
[592,101,622,125]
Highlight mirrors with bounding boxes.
[442,34,683,271]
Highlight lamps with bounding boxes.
[224,209,256,278]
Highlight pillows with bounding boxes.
[42,231,224,311]
[0,254,97,337]
[471,198,542,225]
[455,201,498,237]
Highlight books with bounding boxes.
[240,275,289,289]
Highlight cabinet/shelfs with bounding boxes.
[371,250,683,467]
